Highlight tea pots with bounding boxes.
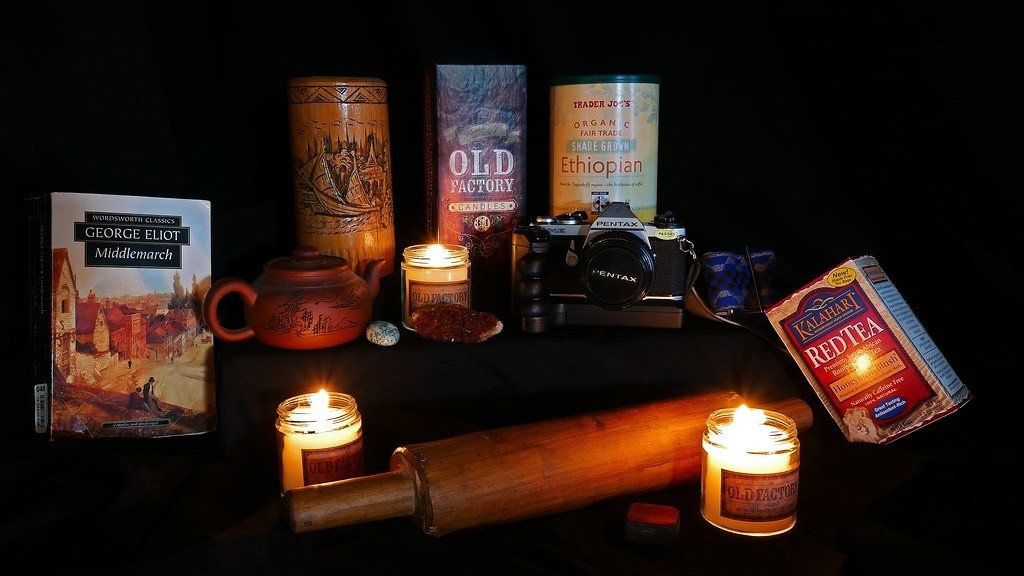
[202,244,388,349]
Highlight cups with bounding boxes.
[274,393,363,496]
[400,243,471,334]
[699,406,799,536]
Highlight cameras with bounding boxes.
[511,202,686,335]
